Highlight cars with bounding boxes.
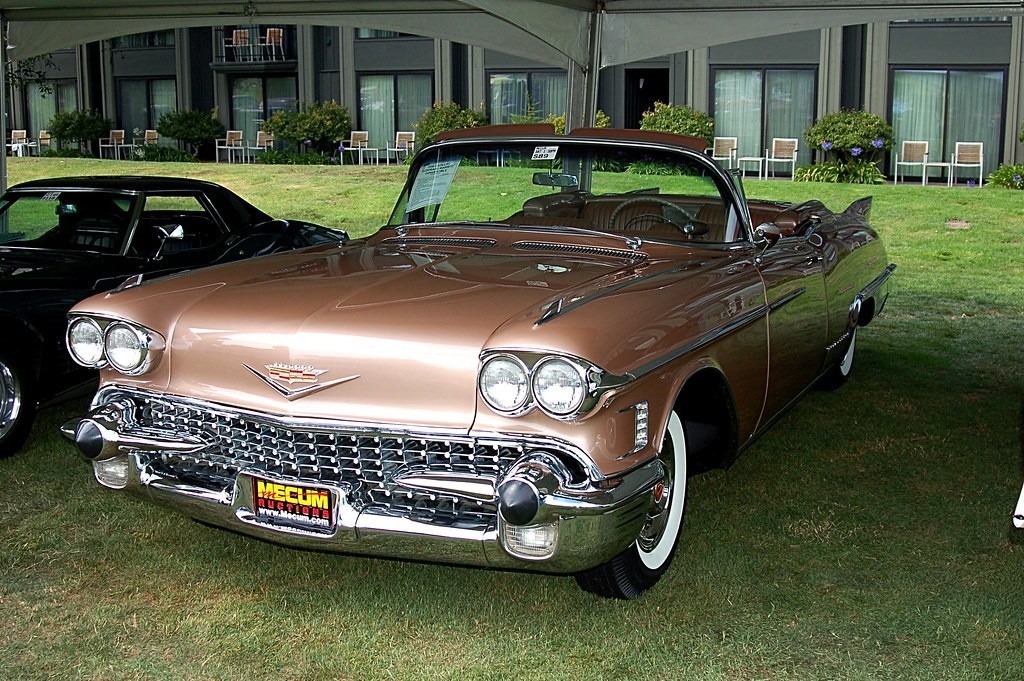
[0,173,350,463]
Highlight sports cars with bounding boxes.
[56,119,897,603]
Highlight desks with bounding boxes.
[737,156,765,180]
[242,44,260,61]
[118,144,133,160]
[12,143,31,156]
[228,147,247,164]
[360,148,391,166]
[926,162,951,187]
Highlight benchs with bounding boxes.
[576,194,786,243]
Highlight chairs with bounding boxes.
[340,131,369,165]
[73,214,215,254]
[765,137,799,180]
[894,141,929,186]
[386,131,416,165]
[215,131,273,164]
[950,141,984,188]
[702,137,738,177]
[98,130,124,160]
[6,130,51,157]
[649,223,726,242]
[476,149,521,168]
[222,28,286,62]
[510,216,594,230]
[132,130,159,150]
